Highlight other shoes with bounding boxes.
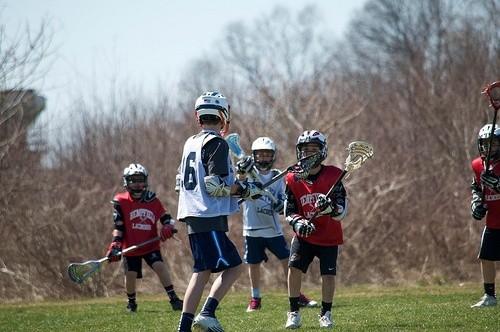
[170,300,183,310]
[127,303,137,312]
[470,293,497,308]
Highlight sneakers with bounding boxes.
[285,311,301,328]
[299,292,317,307]
[318,311,334,328]
[178,313,225,332]
[246,297,261,313]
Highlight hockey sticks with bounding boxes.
[68,229,179,284]
[224,132,324,205]
[307,140,375,226]
[480,81,500,205]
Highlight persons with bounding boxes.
[174,92,251,332]
[471,123,500,308]
[235,137,318,313]
[283,130,348,329]
[106,164,184,313]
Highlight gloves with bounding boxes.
[105,236,122,264]
[316,194,339,215]
[288,213,316,238]
[273,200,284,215]
[160,221,174,242]
[237,156,255,176]
[236,180,267,202]
[480,170,500,191]
[471,200,488,220]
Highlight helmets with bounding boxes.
[123,164,148,187]
[195,92,228,127]
[296,129,328,156]
[251,137,276,162]
[478,123,500,140]
